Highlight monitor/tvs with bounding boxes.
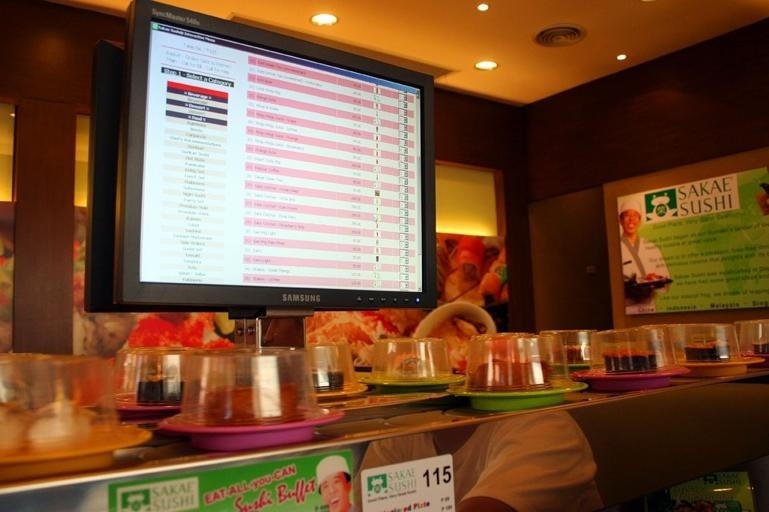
[83,42,380,350]
[114,1,435,346]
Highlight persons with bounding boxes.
[317,455,356,512]
[619,199,668,314]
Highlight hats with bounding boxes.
[619,200,643,218]
[316,455,352,495]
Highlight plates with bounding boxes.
[1,349,768,477]
